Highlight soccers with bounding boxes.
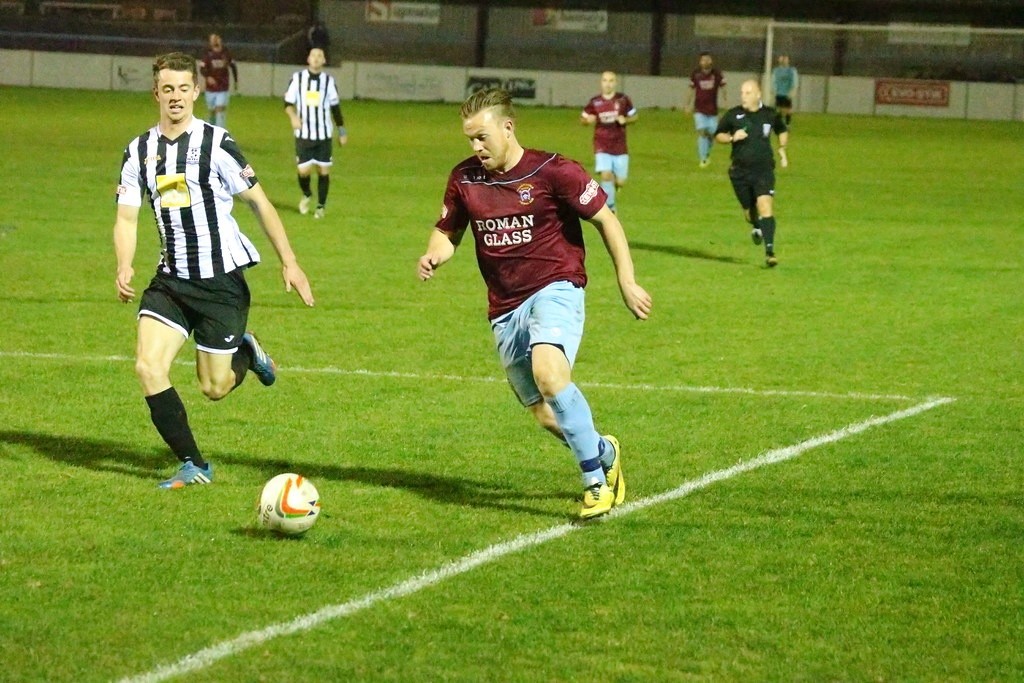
[259,472,321,537]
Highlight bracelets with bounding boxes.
[730,135,733,143]
[338,128,345,136]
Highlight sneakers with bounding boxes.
[298,195,311,214]
[243,330,277,386]
[158,461,212,489]
[579,482,616,519]
[314,208,324,219]
[603,435,626,505]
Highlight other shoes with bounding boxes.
[699,159,710,169]
[612,210,617,216]
[615,181,623,192]
[751,216,763,246]
[706,154,710,160]
[765,252,777,267]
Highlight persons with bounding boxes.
[282,47,348,220]
[417,89,652,521]
[304,19,329,54]
[685,51,727,169]
[772,54,799,126]
[580,71,638,217]
[199,31,239,129]
[114,52,315,489]
[714,79,790,267]
